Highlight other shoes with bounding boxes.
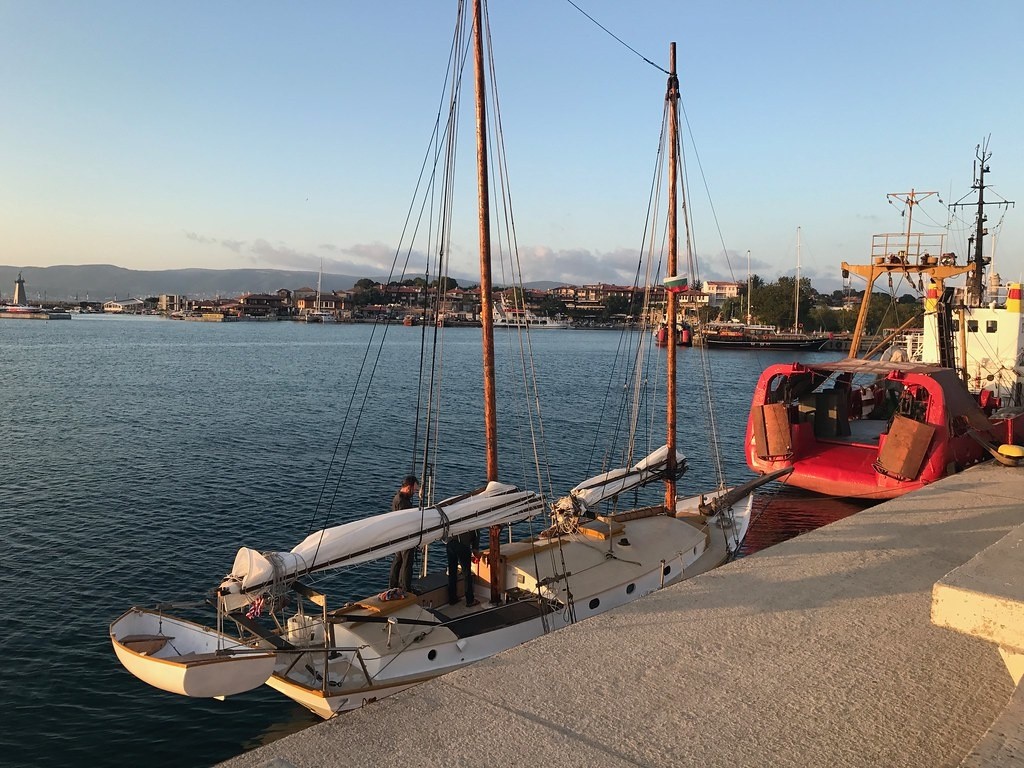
[410,590,422,594]
[449,598,462,605]
[466,599,480,607]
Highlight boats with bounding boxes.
[106,604,279,703]
[490,307,568,331]
[741,134,1023,511]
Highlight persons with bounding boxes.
[388,476,422,593]
[446,530,479,606]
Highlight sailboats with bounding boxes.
[296,256,339,322]
[201,0,803,725]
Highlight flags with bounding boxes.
[658,271,688,293]
